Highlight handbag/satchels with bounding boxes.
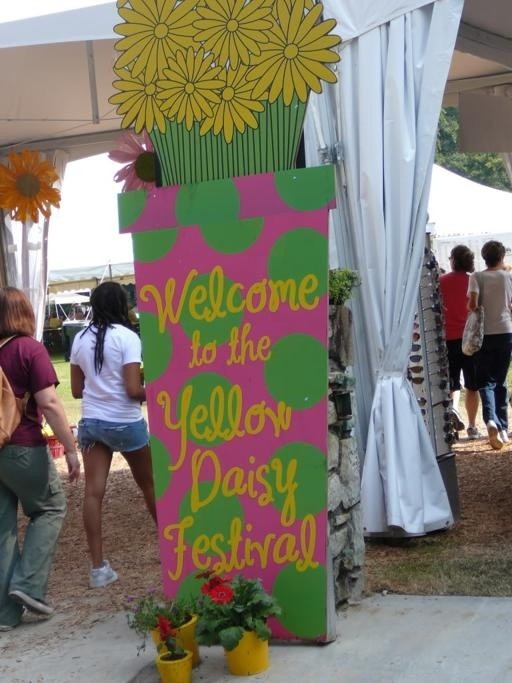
[461,272,486,356]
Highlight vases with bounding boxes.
[147,648,194,682]
[215,617,272,677]
[148,610,202,668]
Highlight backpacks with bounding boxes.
[0,334,31,450]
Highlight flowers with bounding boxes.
[103,0,349,188]
[106,126,165,195]
[188,562,284,654]
[121,582,201,659]
[154,611,188,661]
[0,144,64,224]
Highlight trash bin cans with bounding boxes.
[62,320,90,362]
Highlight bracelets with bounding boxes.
[63,440,79,456]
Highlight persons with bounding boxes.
[466,238,512,451]
[70,283,161,586]
[64,303,84,363]
[84,305,91,319]
[2,285,80,631]
[437,245,488,440]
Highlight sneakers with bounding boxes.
[8,590,54,616]
[487,419,503,449]
[89,560,118,587]
[467,426,482,439]
[501,430,509,443]
[0,608,26,632]
[451,408,465,431]
